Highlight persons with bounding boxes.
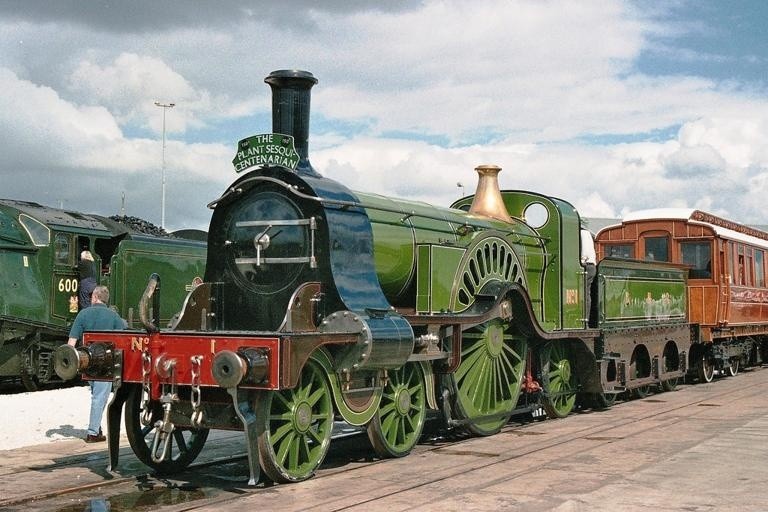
[78,250,97,308]
[68,285,128,442]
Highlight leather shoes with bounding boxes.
[87,428,106,443]
